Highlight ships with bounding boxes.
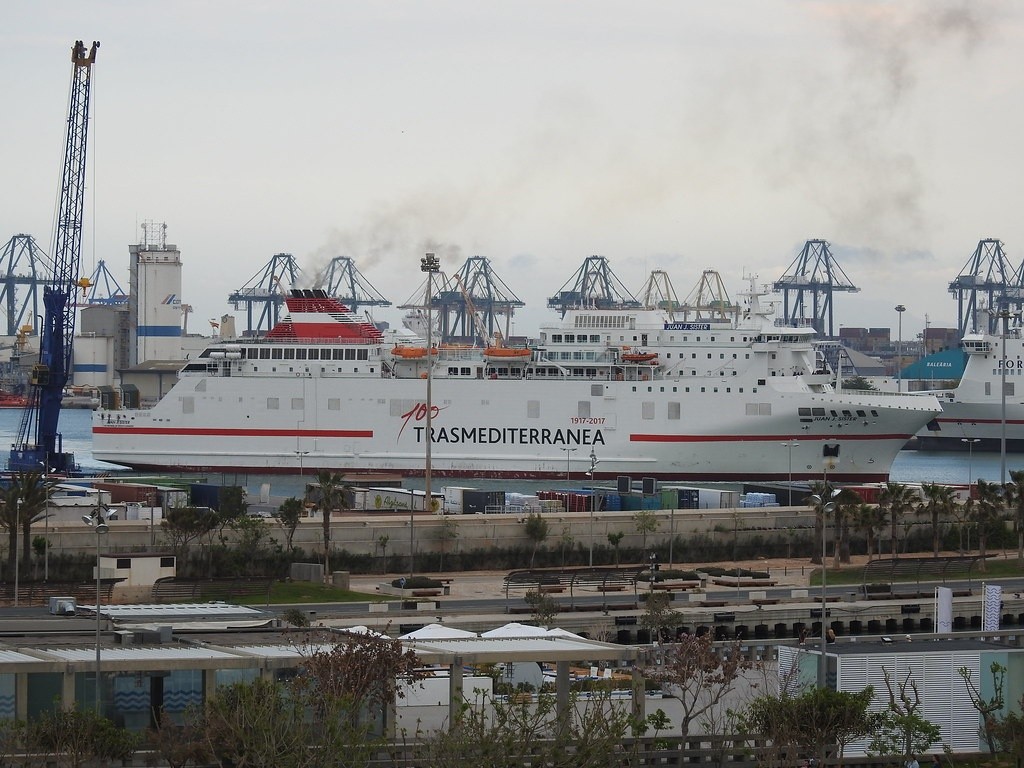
[91,288,944,485]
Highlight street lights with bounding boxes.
[559,447,577,513]
[585,448,600,567]
[986,308,1023,486]
[420,250,442,513]
[894,304,907,392]
[293,450,310,478]
[809,469,842,691]
[961,439,981,498]
[81,482,119,720]
[780,442,799,506]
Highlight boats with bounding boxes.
[482,348,531,362]
[914,310,1024,455]
[621,353,657,361]
[390,346,439,361]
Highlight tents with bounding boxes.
[481,622,586,639]
[340,626,391,639]
[398,624,477,640]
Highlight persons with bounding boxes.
[826,629,835,643]
[798,627,806,643]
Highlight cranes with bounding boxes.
[0,37,130,472]
[227,252,393,339]
[547,237,863,341]
[948,239,1024,346]
[395,256,526,347]
